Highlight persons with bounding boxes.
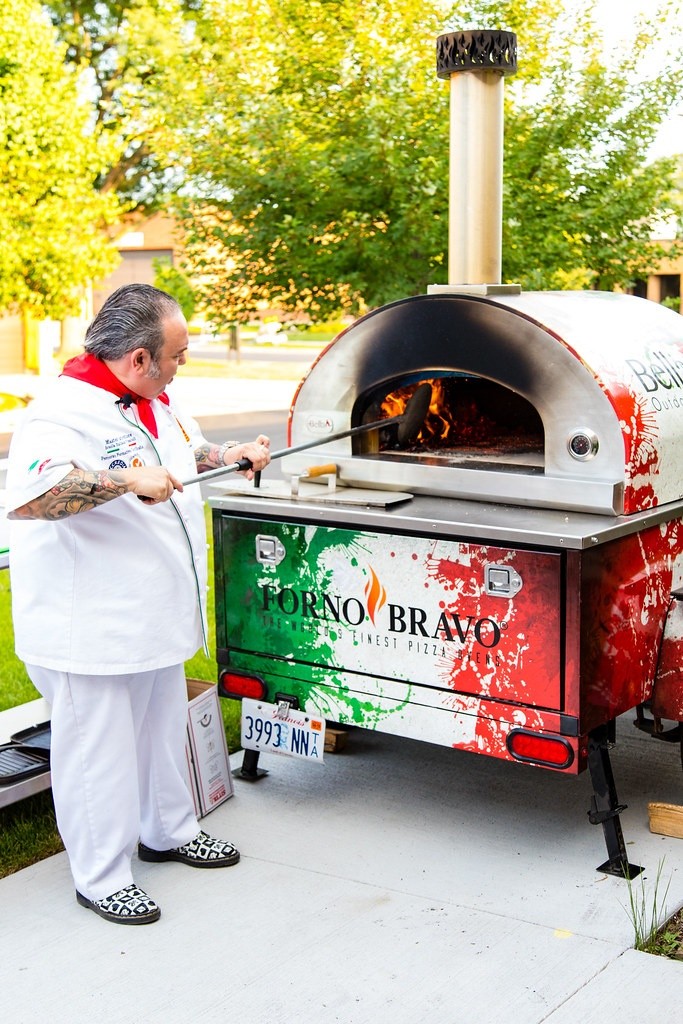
[6,283,271,925]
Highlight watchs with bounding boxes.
[218,440,240,467]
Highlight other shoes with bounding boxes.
[139,830,240,868]
[76,884,161,924]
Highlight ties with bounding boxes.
[56,350,170,439]
